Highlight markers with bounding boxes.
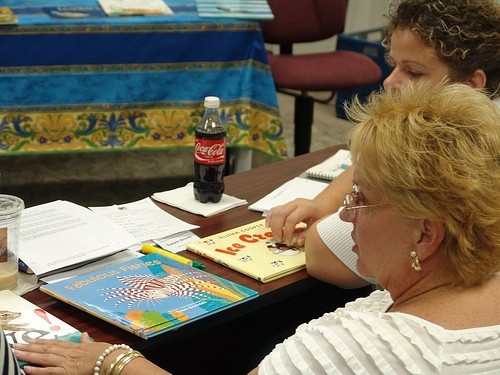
[141,244,207,269]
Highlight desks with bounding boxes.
[0,0,351,348]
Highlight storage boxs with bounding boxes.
[335,28,394,123]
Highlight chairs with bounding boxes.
[258,0,382,157]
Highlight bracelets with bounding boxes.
[106,350,144,375]
[94,344,133,375]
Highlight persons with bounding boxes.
[11,74,500,375]
[266,0,500,290]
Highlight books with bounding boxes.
[39,252,260,340]
[8,247,129,278]
[306,148,351,180]
[187,217,307,283]
[0,290,95,369]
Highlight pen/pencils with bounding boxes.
[338,163,350,168]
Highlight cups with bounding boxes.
[0,194,27,291]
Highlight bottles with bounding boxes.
[193,95,226,203]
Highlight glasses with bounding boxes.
[342,183,398,217]
